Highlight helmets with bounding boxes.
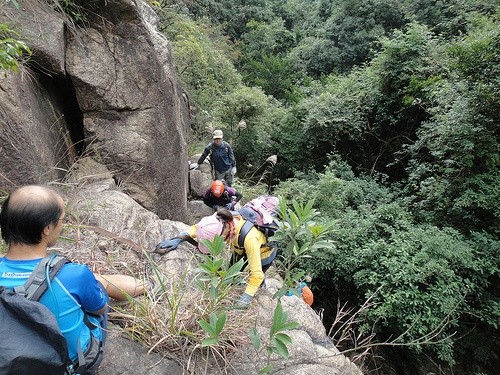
[211,180,224,197]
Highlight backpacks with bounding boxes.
[0,254,69,375]
[236,195,280,248]
[301,286,314,306]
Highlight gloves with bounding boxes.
[226,203,233,210]
[213,204,223,210]
[235,292,253,315]
[231,166,237,176]
[190,163,199,169]
[156,231,190,252]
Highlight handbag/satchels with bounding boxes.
[67,333,104,375]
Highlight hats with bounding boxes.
[212,130,223,139]
[196,216,223,254]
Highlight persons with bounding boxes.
[204,180,243,211]
[0,186,154,375]
[190,129,237,188]
[154,209,314,310]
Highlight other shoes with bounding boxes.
[147,275,165,303]
